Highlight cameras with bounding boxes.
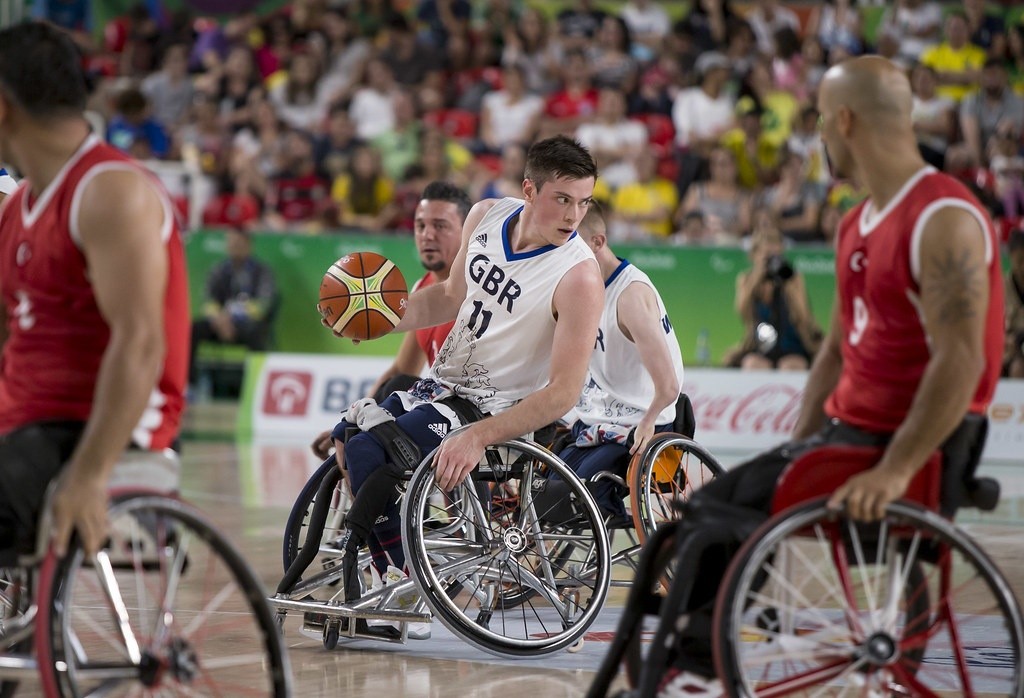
[763,255,794,281]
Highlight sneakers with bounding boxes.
[612,662,727,698]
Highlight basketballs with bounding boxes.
[317,250,411,341]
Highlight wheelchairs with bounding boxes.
[0,440,294,698]
[584,417,1024,698]
[266,394,730,661]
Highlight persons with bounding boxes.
[1004,235,1024,378]
[656,55,1004,696]
[0,18,192,567]
[544,200,685,519]
[717,234,825,372]
[0,0,1021,235]
[190,226,283,391]
[332,132,607,590]
[312,178,473,460]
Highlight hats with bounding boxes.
[693,48,731,76]
